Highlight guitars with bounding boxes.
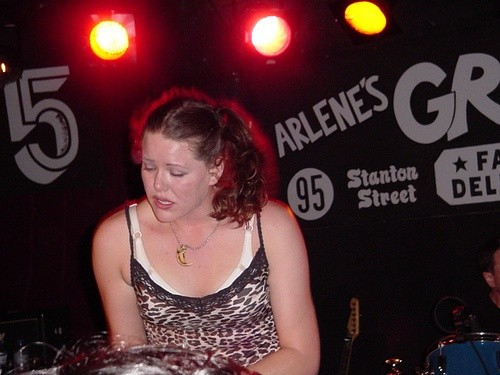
[338,297,363,375]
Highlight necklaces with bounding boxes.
[169,215,221,267]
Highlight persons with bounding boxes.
[464,242,500,333]
[93,88,321,375]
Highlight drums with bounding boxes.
[466,332,500,342]
[432,296,470,336]
